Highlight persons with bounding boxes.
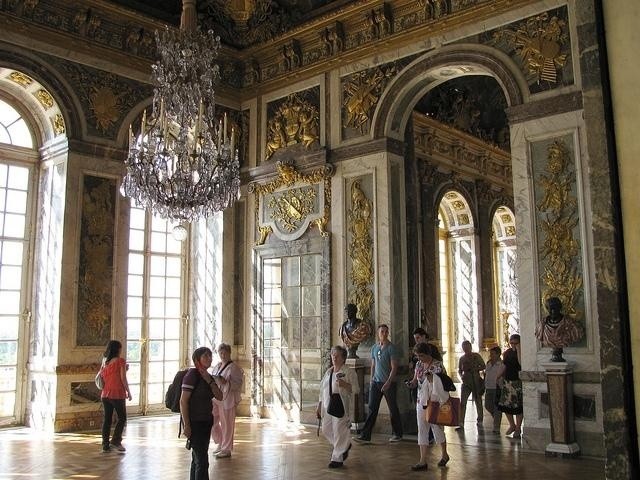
[315,346,361,468]
[454,340,487,434]
[409,327,448,446]
[212,343,244,458]
[299,108,318,149]
[266,119,287,158]
[177,347,223,480]
[99,340,134,454]
[405,341,461,471]
[500,334,523,439]
[350,324,404,444]
[536,296,587,363]
[339,303,369,359]
[483,346,506,433]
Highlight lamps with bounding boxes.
[118,0,245,243]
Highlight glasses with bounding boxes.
[378,351,381,359]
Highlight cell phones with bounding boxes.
[404,380,412,386]
[195,360,204,370]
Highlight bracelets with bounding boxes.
[208,380,214,386]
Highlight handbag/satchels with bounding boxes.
[328,393,344,418]
[425,395,460,427]
[95,373,104,390]
[165,368,201,412]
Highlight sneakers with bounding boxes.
[109,440,125,451]
[328,421,521,471]
[213,444,231,458]
[103,445,110,453]
